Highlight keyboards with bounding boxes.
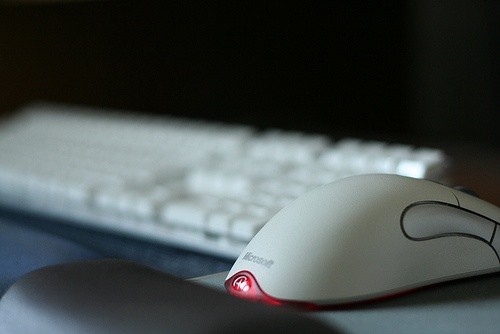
[0,101,450,266]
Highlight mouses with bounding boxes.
[224,172,496,311]
